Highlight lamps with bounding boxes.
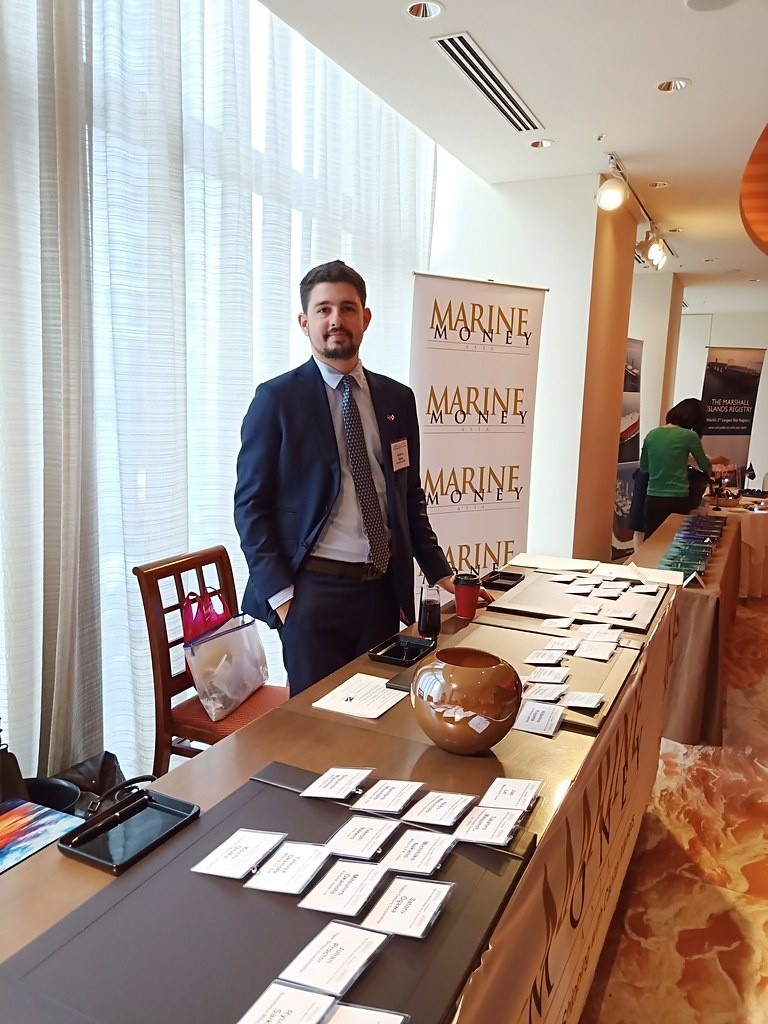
[593,155,630,212]
[635,222,667,270]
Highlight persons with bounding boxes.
[639,398,713,542]
[688,450,730,465]
[234,259,496,699]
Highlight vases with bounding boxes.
[410,647,522,755]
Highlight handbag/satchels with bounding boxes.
[72,776,156,818]
[181,586,269,723]
[688,464,709,510]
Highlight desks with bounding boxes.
[623,514,741,747]
[690,486,768,599]
[0,553,691,1024]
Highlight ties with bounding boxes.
[339,375,392,575]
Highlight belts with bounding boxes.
[301,557,391,581]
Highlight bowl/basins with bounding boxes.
[22,777,81,816]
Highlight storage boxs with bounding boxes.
[657,514,728,583]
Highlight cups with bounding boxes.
[417,584,441,640]
[453,573,482,619]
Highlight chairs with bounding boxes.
[132,545,289,782]
